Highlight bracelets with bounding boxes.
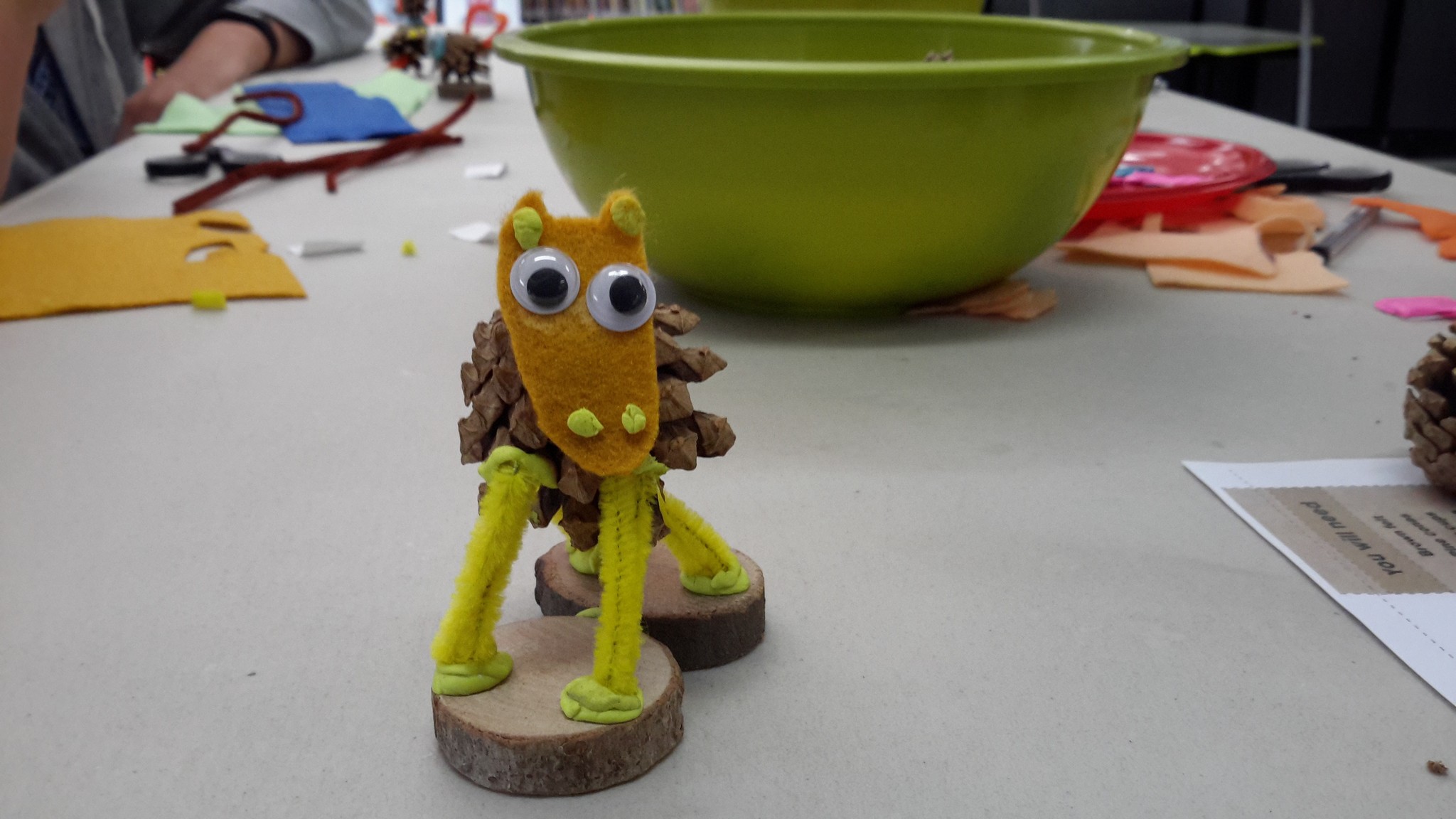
[220,2,280,69]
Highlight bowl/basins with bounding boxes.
[491,10,1189,314]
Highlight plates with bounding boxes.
[1082,133,1277,221]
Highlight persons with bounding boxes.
[0,0,375,207]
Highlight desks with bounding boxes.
[0,23,1456,819]
